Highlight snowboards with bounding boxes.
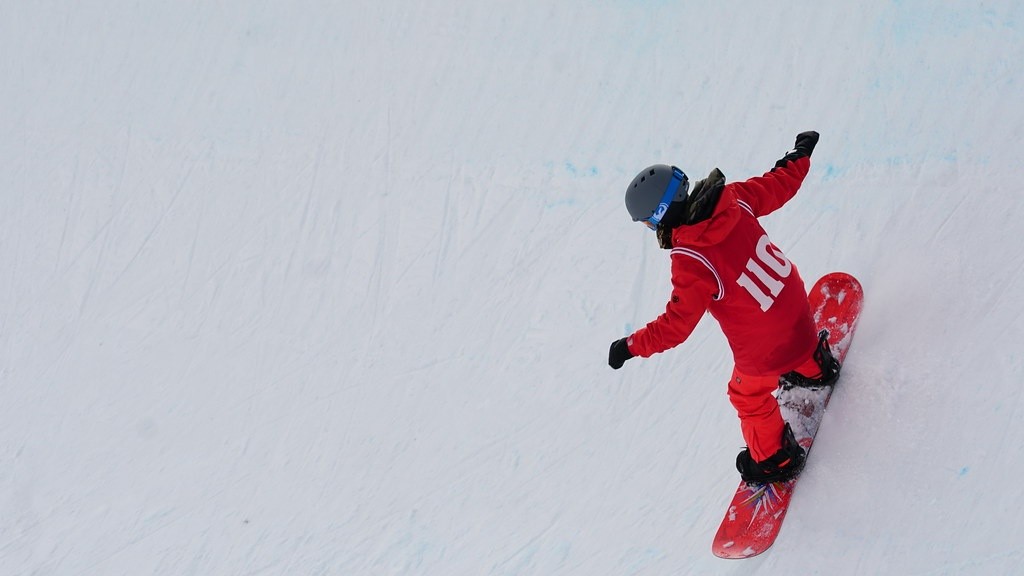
[705,272,868,559]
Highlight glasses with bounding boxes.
[643,165,685,231]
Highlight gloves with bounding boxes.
[608,336,635,370]
[793,130,820,158]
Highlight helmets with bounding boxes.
[625,164,689,222]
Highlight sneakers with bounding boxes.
[735,422,806,483]
[780,329,841,391]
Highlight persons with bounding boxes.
[608,131,840,483]
